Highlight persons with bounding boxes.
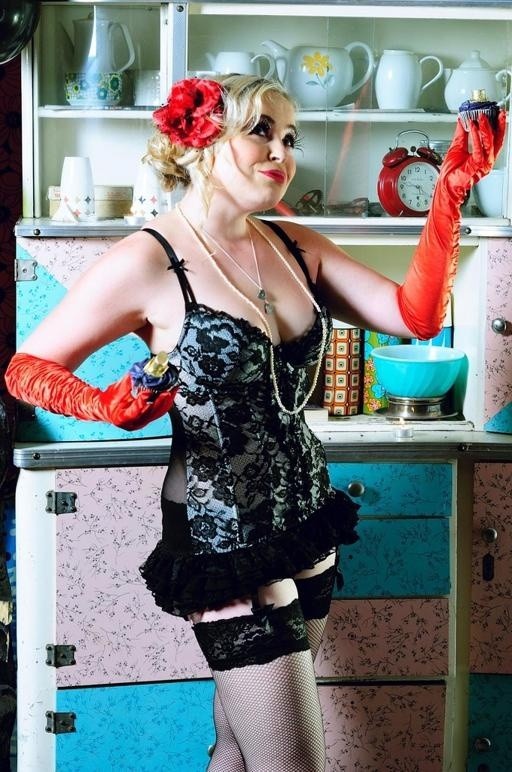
[6,77,505,772]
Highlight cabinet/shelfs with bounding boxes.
[16,411,511,772]
[15,0,512,441]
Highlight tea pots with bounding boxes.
[53,11,134,107]
[258,39,375,114]
[205,50,275,82]
[441,50,511,115]
[375,49,443,109]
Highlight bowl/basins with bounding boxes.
[48,185,133,218]
[370,344,466,398]
[123,68,161,107]
[472,171,507,215]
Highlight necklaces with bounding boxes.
[178,202,274,315]
[176,201,336,416]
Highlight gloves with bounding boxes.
[397,111,507,340]
[5,355,178,432]
